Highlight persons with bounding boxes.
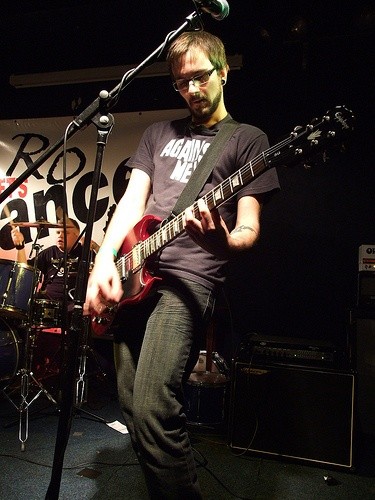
[83,31,282,500]
[10,218,96,303]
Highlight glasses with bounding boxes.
[173,65,217,92]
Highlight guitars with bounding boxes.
[89,104,357,335]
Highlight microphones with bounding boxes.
[195,0,229,21]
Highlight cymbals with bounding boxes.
[7,219,75,228]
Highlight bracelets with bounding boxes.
[98,246,117,257]
[15,240,25,250]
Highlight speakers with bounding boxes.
[228,358,358,470]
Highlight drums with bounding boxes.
[0,258,45,320]
[185,371,230,426]
[0,317,20,391]
[31,299,64,328]
[192,350,231,374]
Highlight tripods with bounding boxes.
[0,227,111,452]
